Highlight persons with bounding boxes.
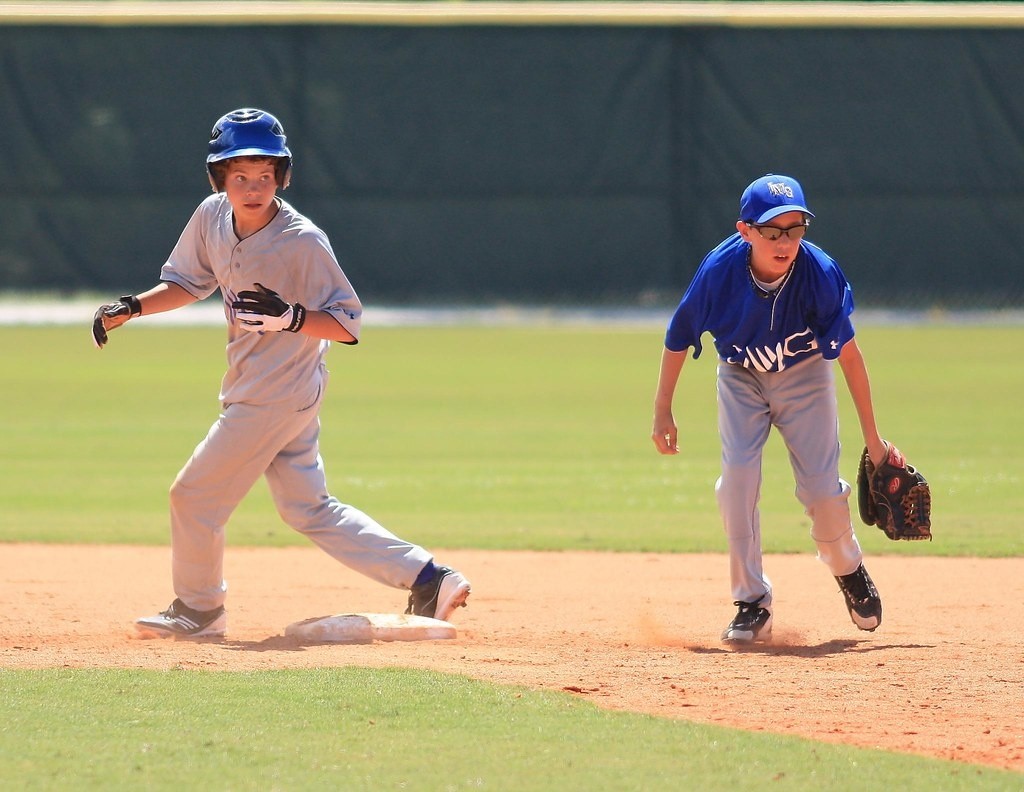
[93,107,471,638]
[652,173,933,648]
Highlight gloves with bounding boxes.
[91,294,142,350]
[231,282,307,334]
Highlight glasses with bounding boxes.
[746,219,810,241]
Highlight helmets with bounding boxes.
[205,108,293,194]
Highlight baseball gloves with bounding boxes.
[856,440,932,542]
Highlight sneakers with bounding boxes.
[720,594,773,644]
[135,598,228,643]
[404,565,471,622]
[835,561,883,632]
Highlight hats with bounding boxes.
[737,173,815,224]
